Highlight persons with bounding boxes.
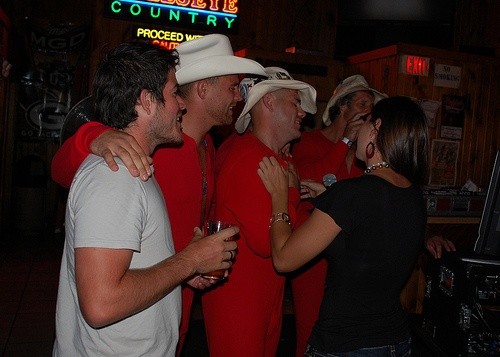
[198,66,316,357]
[257,96,430,357]
[51,33,270,357]
[289,75,456,357]
[51,40,239,357]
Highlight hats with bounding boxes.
[235,66,318,134]
[323,75,388,128]
[172,34,269,85]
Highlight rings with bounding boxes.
[230,251,233,260]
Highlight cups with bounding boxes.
[200,220,233,280]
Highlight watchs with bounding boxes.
[269,213,291,226]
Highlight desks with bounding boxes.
[412,207,483,314]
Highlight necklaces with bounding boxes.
[362,161,391,174]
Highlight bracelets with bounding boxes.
[342,136,354,148]
